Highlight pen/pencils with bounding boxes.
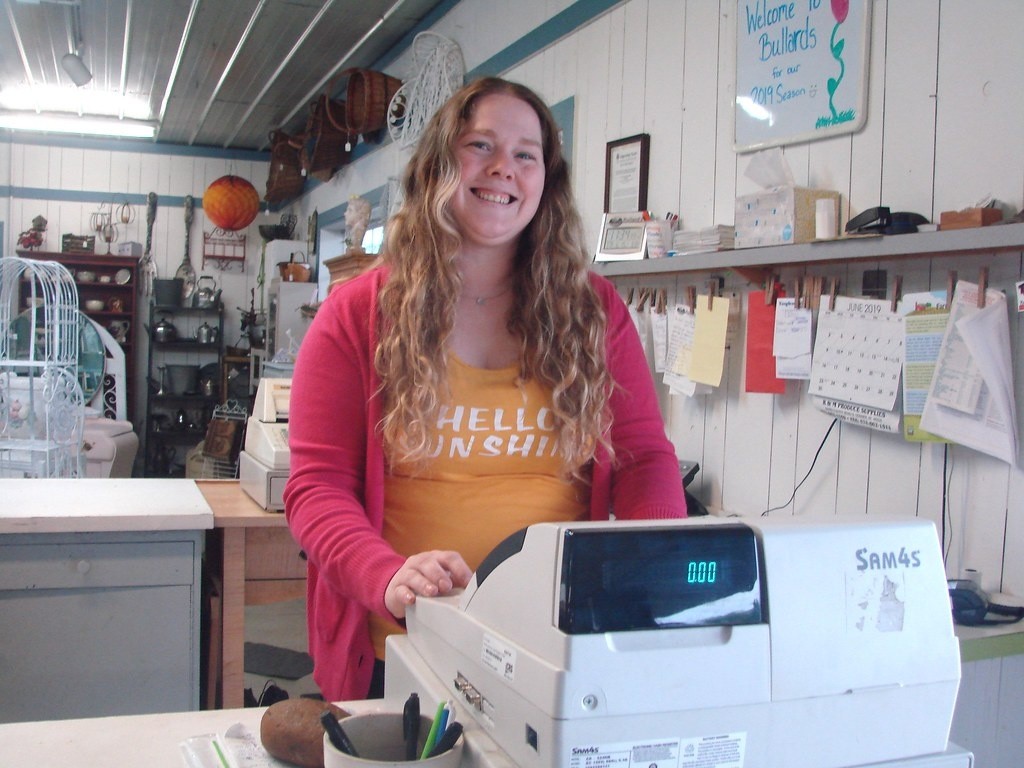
[665,212,678,221]
[420,699,456,760]
[642,211,655,221]
[403,692,420,760]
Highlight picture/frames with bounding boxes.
[602,134,650,214]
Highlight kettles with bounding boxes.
[193,275,223,308]
[200,375,218,397]
[143,318,177,342]
[191,321,220,345]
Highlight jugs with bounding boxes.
[108,320,130,343]
[153,444,176,476]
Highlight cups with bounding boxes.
[646,219,673,257]
[815,198,836,239]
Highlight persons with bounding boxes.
[344,198,370,247]
[282,75,688,705]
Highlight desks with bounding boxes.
[1,695,388,766]
[197,477,311,712]
[1,477,214,719]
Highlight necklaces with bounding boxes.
[459,287,510,306]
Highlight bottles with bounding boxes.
[323,712,465,767]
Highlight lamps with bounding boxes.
[61,53,94,88]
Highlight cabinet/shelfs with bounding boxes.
[144,299,230,455]
[16,246,135,387]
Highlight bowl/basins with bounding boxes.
[98,276,111,283]
[85,300,105,310]
[27,298,44,308]
[76,271,96,283]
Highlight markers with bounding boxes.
[320,712,360,757]
[426,721,463,759]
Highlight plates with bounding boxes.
[115,268,131,284]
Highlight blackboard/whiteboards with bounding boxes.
[736,0,873,153]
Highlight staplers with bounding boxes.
[844,206,892,234]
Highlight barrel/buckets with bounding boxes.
[153,277,184,310]
[167,364,200,393]
